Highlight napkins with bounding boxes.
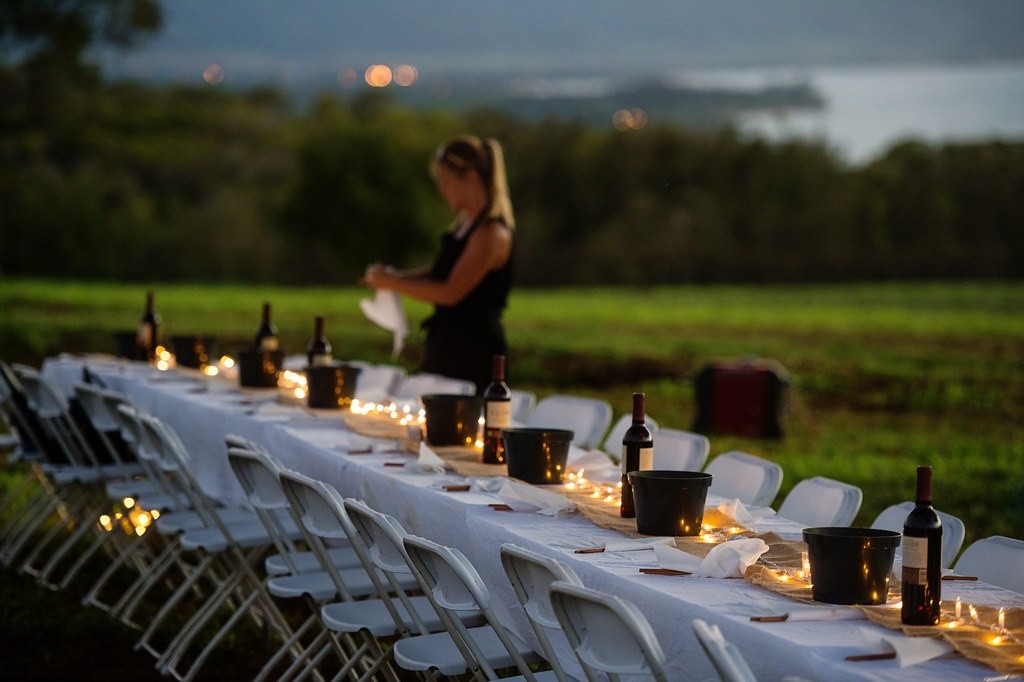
[653,537,770,581]
[385,440,448,475]
[876,632,962,668]
[781,605,864,623]
[465,473,504,494]
[358,274,412,361]
[496,479,576,518]
[717,499,786,526]
[572,448,620,484]
[368,444,399,452]
[891,562,955,584]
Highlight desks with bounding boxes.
[44,356,1024,680]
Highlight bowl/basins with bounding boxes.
[421,394,482,447]
[302,366,362,409]
[802,526,901,606]
[627,470,713,537]
[237,350,285,387]
[169,335,215,369]
[501,428,574,486]
[110,331,148,361]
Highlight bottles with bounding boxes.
[253,302,280,352]
[137,293,157,359]
[900,466,944,626]
[482,356,512,465]
[305,316,333,368]
[621,391,654,519]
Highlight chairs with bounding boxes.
[1,343,1024,682]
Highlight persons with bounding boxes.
[356,133,518,402]
